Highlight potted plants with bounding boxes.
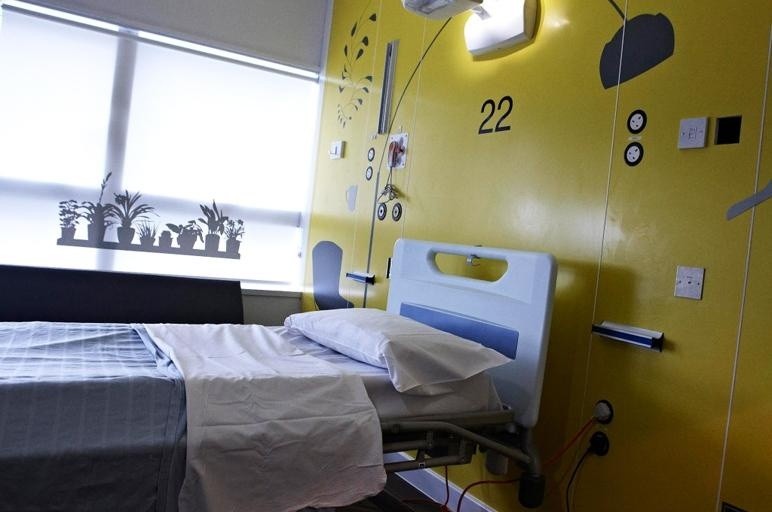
[58,172,245,253]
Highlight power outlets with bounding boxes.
[590,432,609,456]
[592,400,616,428]
[401,0,538,57]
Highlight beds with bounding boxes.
[0,237,557,512]
